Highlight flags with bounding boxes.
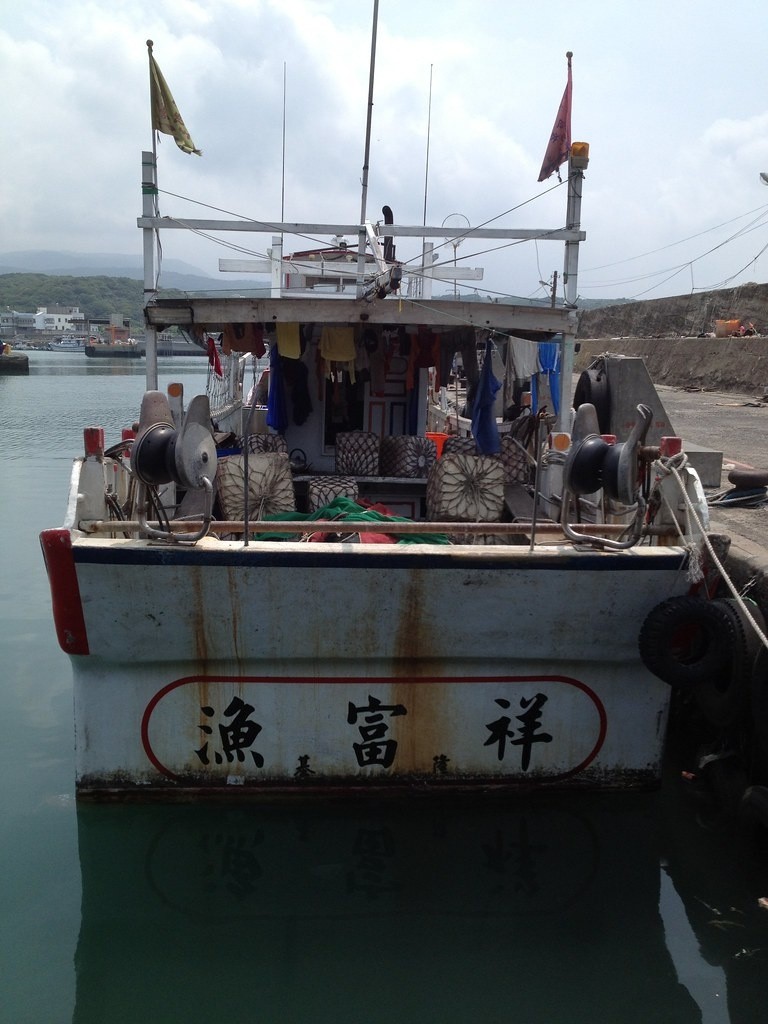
[149,48,203,157]
[538,68,572,181]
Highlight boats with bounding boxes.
[39,0,732,804]
[48,332,87,352]
[282,234,406,283]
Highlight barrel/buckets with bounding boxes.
[426,433,449,458]
